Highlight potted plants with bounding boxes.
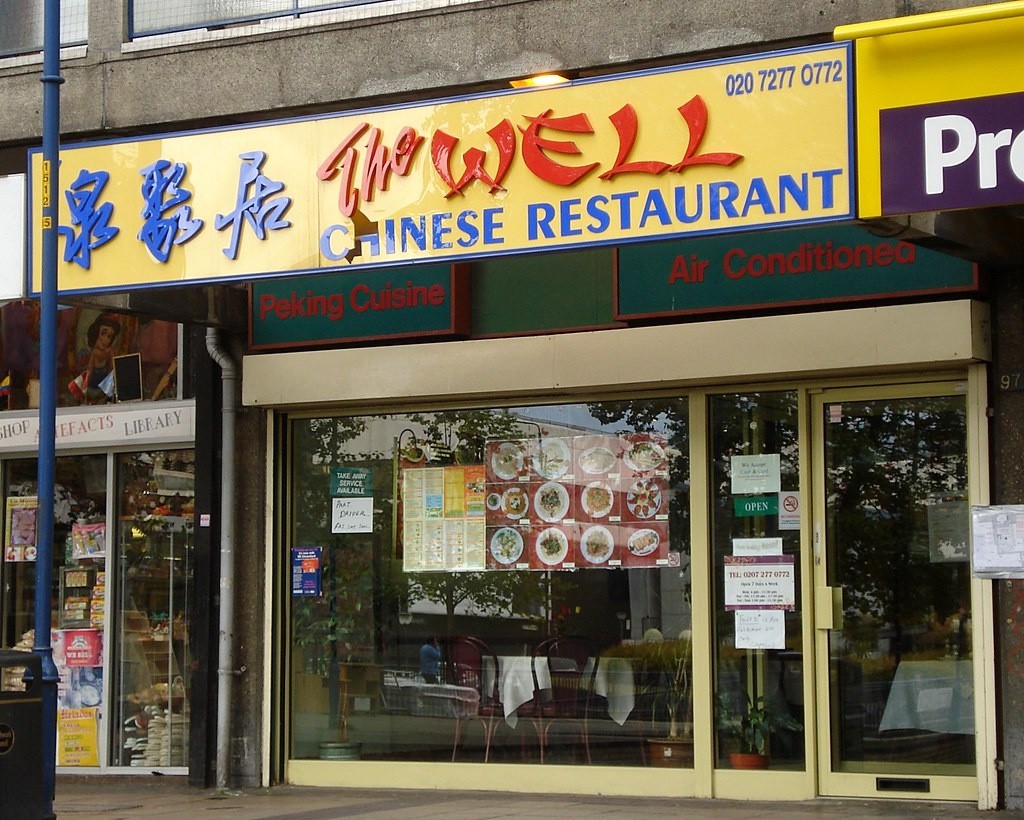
[156,458,193,489]
[292,542,390,761]
[638,615,693,769]
[714,685,803,771]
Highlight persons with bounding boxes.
[422,635,441,686]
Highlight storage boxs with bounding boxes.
[62,630,103,666]
[120,515,195,768]
[72,522,105,559]
[64,572,106,629]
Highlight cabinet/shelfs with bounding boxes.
[58,565,98,629]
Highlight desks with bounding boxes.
[478,656,636,766]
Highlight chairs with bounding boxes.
[454,634,601,765]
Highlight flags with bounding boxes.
[0,376,10,396]
[97,371,115,397]
[68,371,88,400]
